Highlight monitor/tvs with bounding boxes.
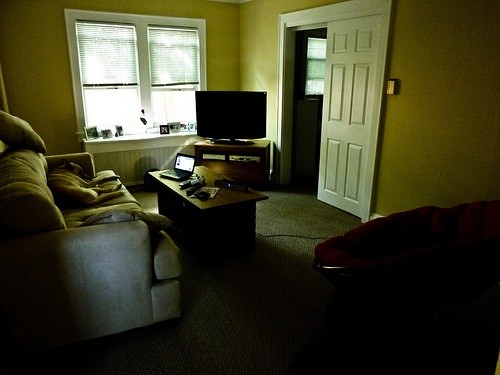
[195,90,267,145]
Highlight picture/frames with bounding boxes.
[101,129,112,139]
[115,126,123,136]
[159,122,196,134]
[84,126,98,140]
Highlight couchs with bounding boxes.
[0,149,183,355]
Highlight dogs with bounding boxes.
[47,159,123,207]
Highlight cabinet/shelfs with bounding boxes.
[193,141,272,191]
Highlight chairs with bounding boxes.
[310,200,500,293]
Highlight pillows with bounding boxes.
[79,210,172,227]
[1,109,46,153]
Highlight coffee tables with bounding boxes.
[148,166,269,253]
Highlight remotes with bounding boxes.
[178,179,202,195]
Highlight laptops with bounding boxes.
[160,151,198,180]
